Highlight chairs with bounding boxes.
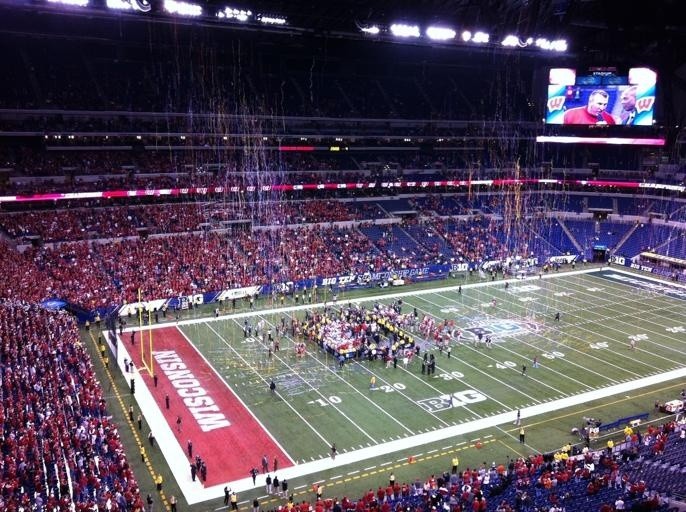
[386,413,686,512]
[317,192,685,276]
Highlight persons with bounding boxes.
[0,305,180,511]
[553,311,561,322]
[618,85,638,126]
[224,486,231,505]
[0,106,686,311]
[212,309,494,390]
[200,460,207,483]
[195,454,202,473]
[270,380,277,394]
[227,389,686,512]
[175,415,183,433]
[627,336,636,352]
[190,461,196,482]
[521,363,527,375]
[532,354,539,370]
[186,438,193,458]
[563,89,617,129]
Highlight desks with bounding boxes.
[665,400,683,412]
